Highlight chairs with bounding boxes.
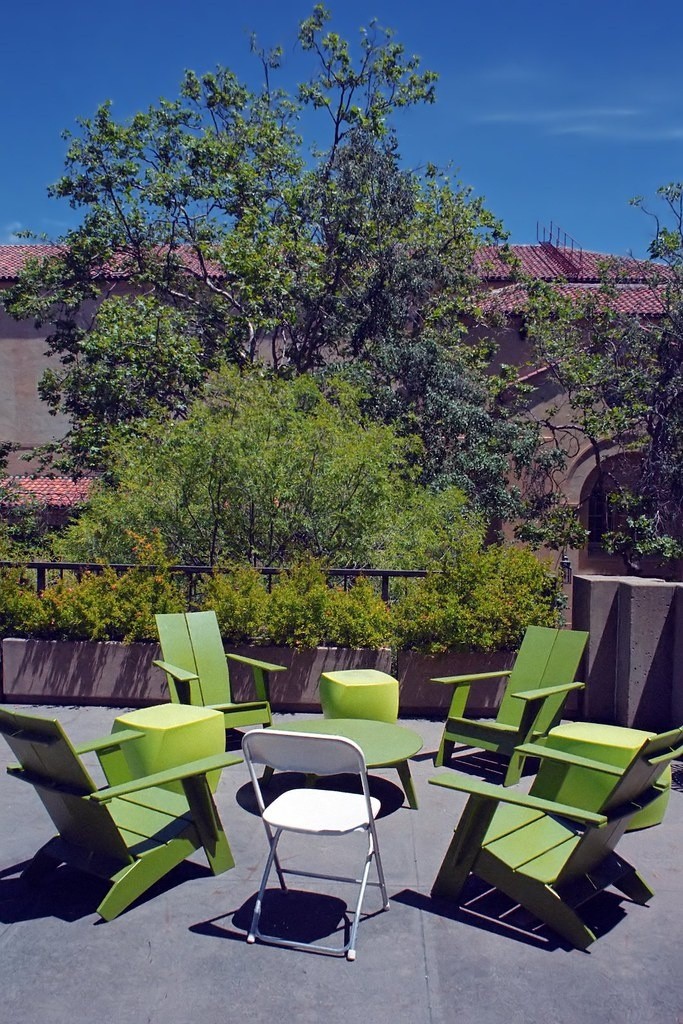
[428,726,683,950]
[152,610,288,729]
[0,708,246,923]
[428,624,590,788]
[242,727,390,960]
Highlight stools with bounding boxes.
[526,722,671,832]
[319,669,399,724]
[113,702,225,796]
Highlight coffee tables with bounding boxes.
[257,718,424,811]
